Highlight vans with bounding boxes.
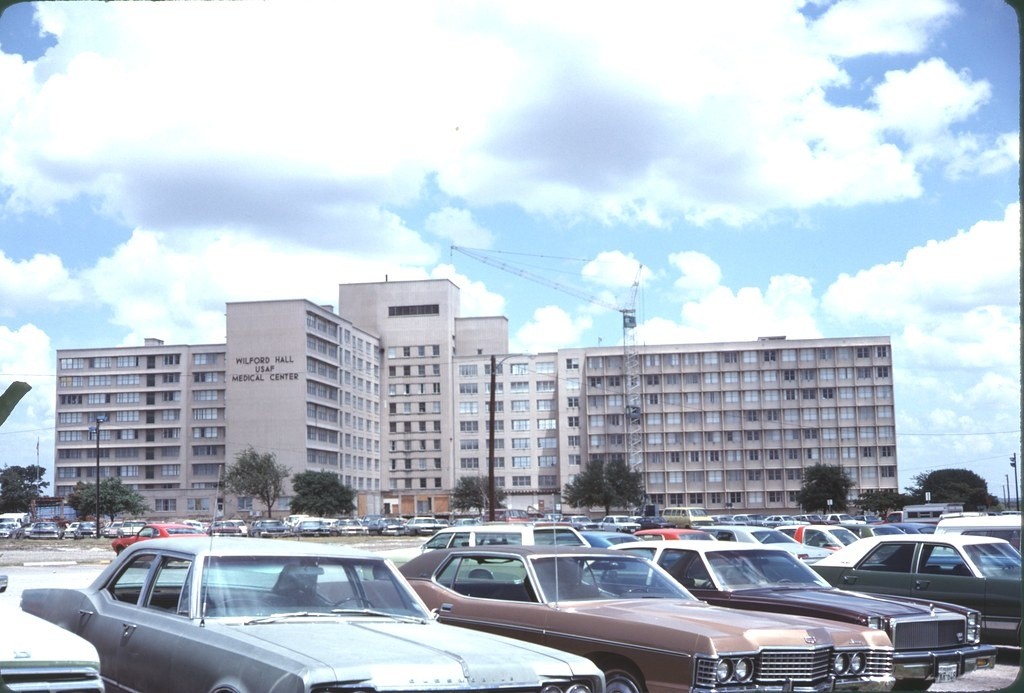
[484,508,530,522]
[662,507,714,529]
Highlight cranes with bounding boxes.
[450,244,648,512]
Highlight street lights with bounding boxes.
[488,351,538,521]
[1010,453,1019,511]
[88,416,107,539]
[1005,474,1010,510]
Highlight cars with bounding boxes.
[602,515,641,533]
[581,540,1000,693]
[792,515,836,525]
[317,545,896,693]
[0,576,106,693]
[558,516,592,524]
[19,536,606,693]
[852,515,883,523]
[636,517,676,529]
[808,535,1024,645]
[934,515,1022,556]
[762,515,810,526]
[411,523,937,565]
[110,524,207,563]
[733,514,763,525]
[822,513,866,525]
[0,513,479,541]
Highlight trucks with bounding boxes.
[882,503,965,524]
